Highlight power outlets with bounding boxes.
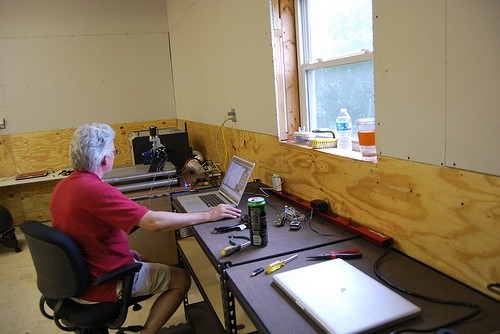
[231,108,236,122]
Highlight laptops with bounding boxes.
[272,257,421,334]
[176,155,255,213]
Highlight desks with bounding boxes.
[170,182,356,334]
[0,167,75,187]
[122,178,222,201]
[225,237,500,334]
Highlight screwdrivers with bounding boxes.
[221,241,251,257]
[265,254,299,274]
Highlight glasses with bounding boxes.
[112,149,119,155]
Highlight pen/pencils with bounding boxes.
[250,266,264,277]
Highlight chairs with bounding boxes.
[20,220,155,334]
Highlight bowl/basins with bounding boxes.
[294,132,316,144]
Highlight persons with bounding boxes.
[50,123,242,334]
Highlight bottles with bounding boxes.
[336,108,352,155]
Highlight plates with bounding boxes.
[16,170,47,179]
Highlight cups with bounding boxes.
[357,118,376,161]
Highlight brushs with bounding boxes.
[311,130,337,149]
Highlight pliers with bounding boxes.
[227,234,250,245]
[305,248,363,259]
